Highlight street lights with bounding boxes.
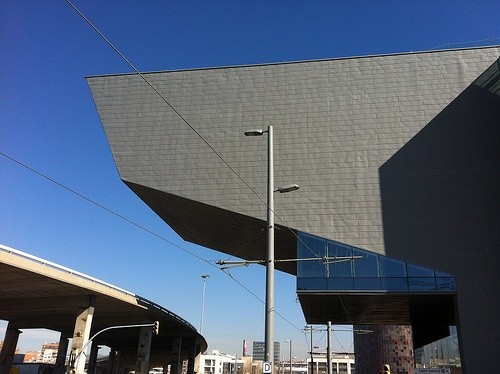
[65,321,159,374]
[214,125,365,374]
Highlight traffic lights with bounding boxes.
[382,364,391,374]
